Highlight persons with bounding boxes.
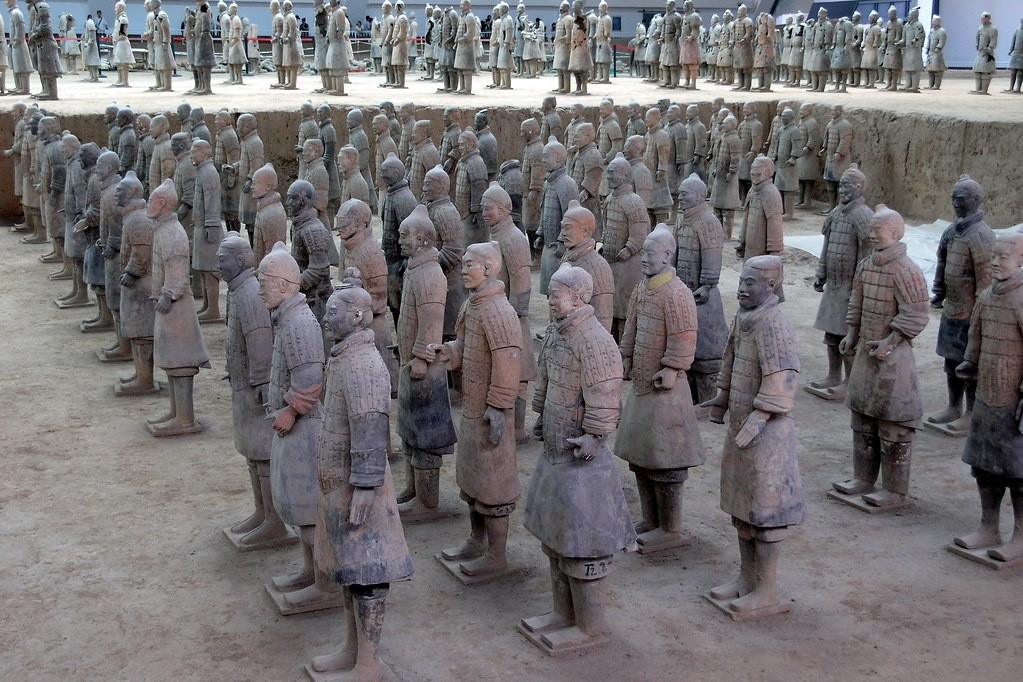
[930,174,1023,562]
[216,15,221,37]
[699,254,807,614]
[523,262,637,652]
[257,242,345,607]
[311,276,413,682]
[94,10,109,37]
[484,14,493,39]
[84,14,92,28]
[3,99,930,585]
[180,10,195,36]
[0,0,1023,99]
[366,16,369,38]
[355,20,364,38]
[210,13,215,38]
[295,14,300,22]
[536,17,548,42]
[551,22,556,42]
[300,17,309,37]
[368,18,373,38]
